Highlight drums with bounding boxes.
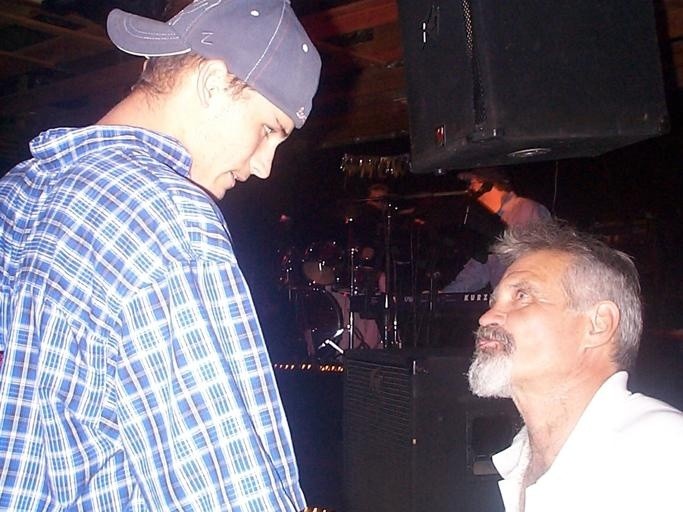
[277,284,381,366]
[303,242,346,286]
[271,244,305,280]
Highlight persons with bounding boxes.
[348,163,554,347]
[468,217,683,512]
[1,1,321,511]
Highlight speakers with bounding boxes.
[339,345,523,512]
[398,0,672,176]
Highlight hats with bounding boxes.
[106,0,322,130]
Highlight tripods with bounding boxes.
[314,265,366,355]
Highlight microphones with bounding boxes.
[464,178,495,208]
